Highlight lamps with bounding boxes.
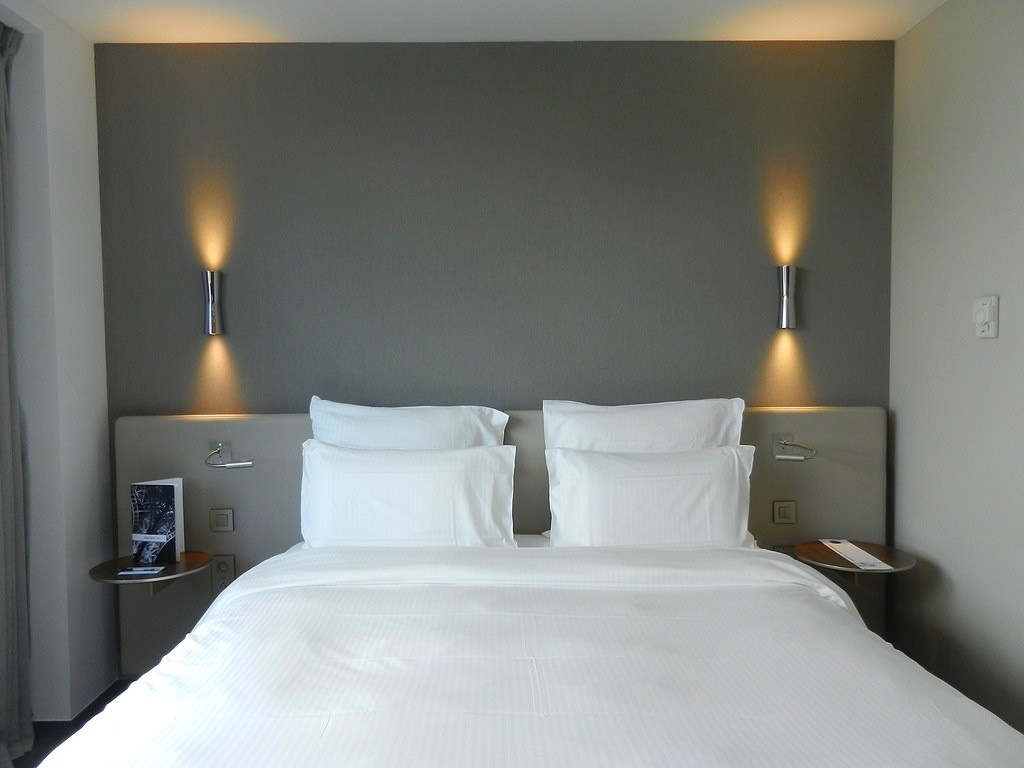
[777,265,799,330]
[204,270,224,336]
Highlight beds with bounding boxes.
[37,408,1023,768]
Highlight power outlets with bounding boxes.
[212,555,235,596]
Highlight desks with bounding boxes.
[773,541,918,593]
[90,552,212,598]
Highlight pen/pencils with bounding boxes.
[115,568,161,571]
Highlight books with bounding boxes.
[130,478,185,566]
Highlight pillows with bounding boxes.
[540,397,745,539]
[545,445,759,551]
[309,396,510,450]
[301,438,518,548]
